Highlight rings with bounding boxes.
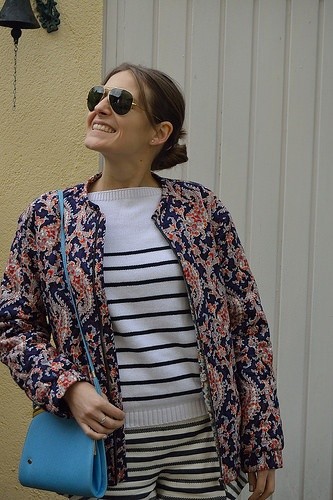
[101,417,106,423]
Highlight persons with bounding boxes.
[0,62,284,500]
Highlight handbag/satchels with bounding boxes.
[18,412,107,498]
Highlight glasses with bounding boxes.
[85,85,162,122]
